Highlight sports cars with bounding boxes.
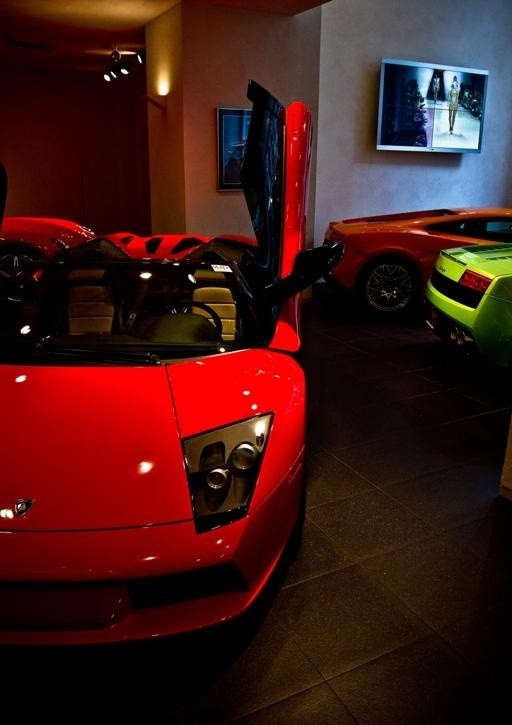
[0,74,344,688]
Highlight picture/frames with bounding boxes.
[217,107,251,192]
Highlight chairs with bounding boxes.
[172,248,246,352]
[39,249,127,336]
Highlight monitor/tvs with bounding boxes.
[375,56,489,154]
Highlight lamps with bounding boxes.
[137,48,145,64]
[98,45,133,83]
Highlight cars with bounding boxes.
[426,245,510,369]
[323,204,512,316]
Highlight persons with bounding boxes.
[432,73,440,104]
[448,75,459,134]
